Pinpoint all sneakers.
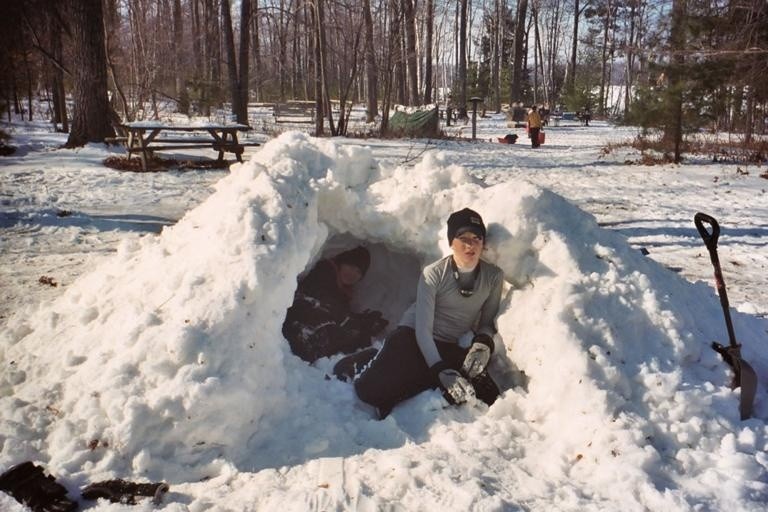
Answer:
[335,346,378,381]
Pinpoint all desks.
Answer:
[110,123,249,172]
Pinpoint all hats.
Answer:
[446,208,487,246]
[335,246,370,276]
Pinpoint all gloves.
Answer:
[353,311,388,341]
[461,335,494,379]
[430,361,477,405]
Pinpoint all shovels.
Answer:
[694,212,758,422]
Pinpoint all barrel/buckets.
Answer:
[538,127,545,143]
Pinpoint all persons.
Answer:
[339,204,522,417]
[585,106,592,126]
[445,96,455,127]
[527,105,543,149]
[281,242,389,359]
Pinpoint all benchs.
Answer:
[104,137,260,172]
